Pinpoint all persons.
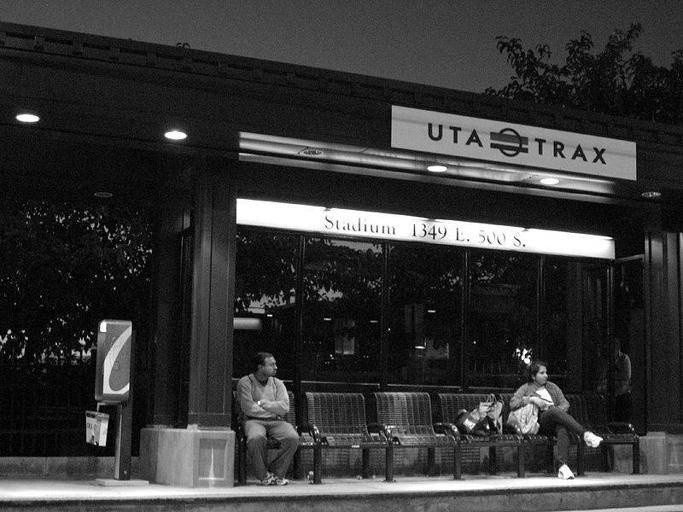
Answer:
[510,360,604,479]
[236,351,300,486]
[606,337,634,425]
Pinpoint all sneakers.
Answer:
[255,472,289,486]
[558,464,575,480]
[584,431,603,448]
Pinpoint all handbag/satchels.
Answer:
[456,393,504,436]
[507,403,540,435]
